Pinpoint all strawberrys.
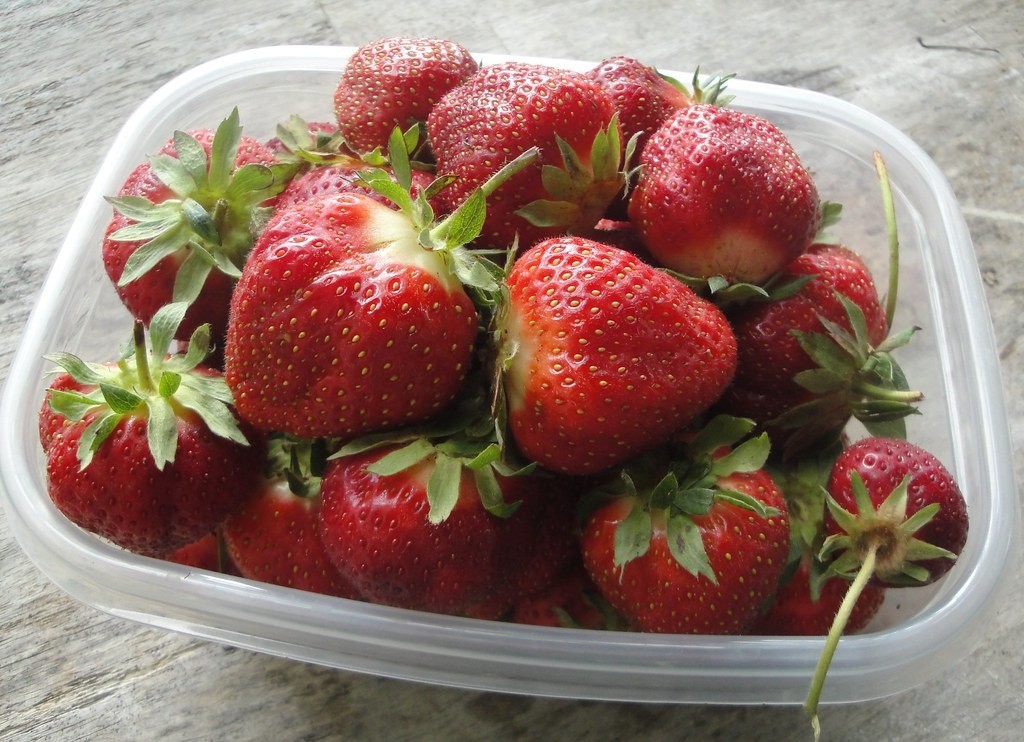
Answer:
[38,36,971,636]
